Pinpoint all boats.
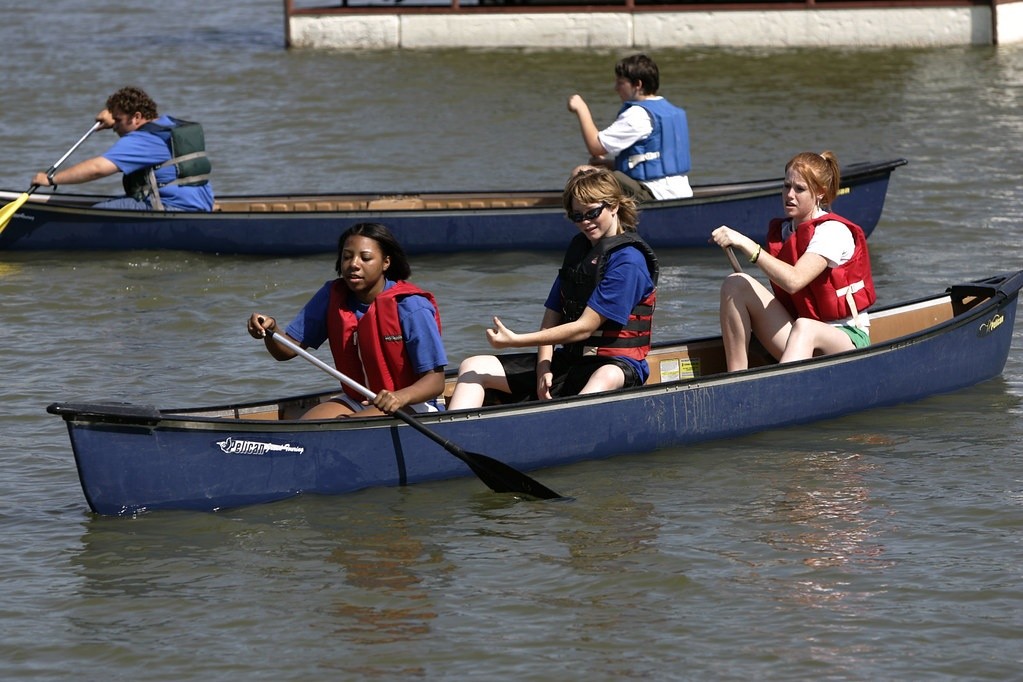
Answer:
[47,270,1023,512]
[1,159,908,254]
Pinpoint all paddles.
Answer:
[0,122,103,234]
[724,246,743,274]
[252,318,558,498]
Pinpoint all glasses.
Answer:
[568,202,607,223]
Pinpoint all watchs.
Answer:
[47,172,58,191]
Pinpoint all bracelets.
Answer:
[750,242,763,265]
[269,316,276,331]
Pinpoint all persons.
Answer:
[568,54,694,203]
[247,223,446,420]
[447,169,661,411]
[30,88,221,213]
[708,150,877,373]
